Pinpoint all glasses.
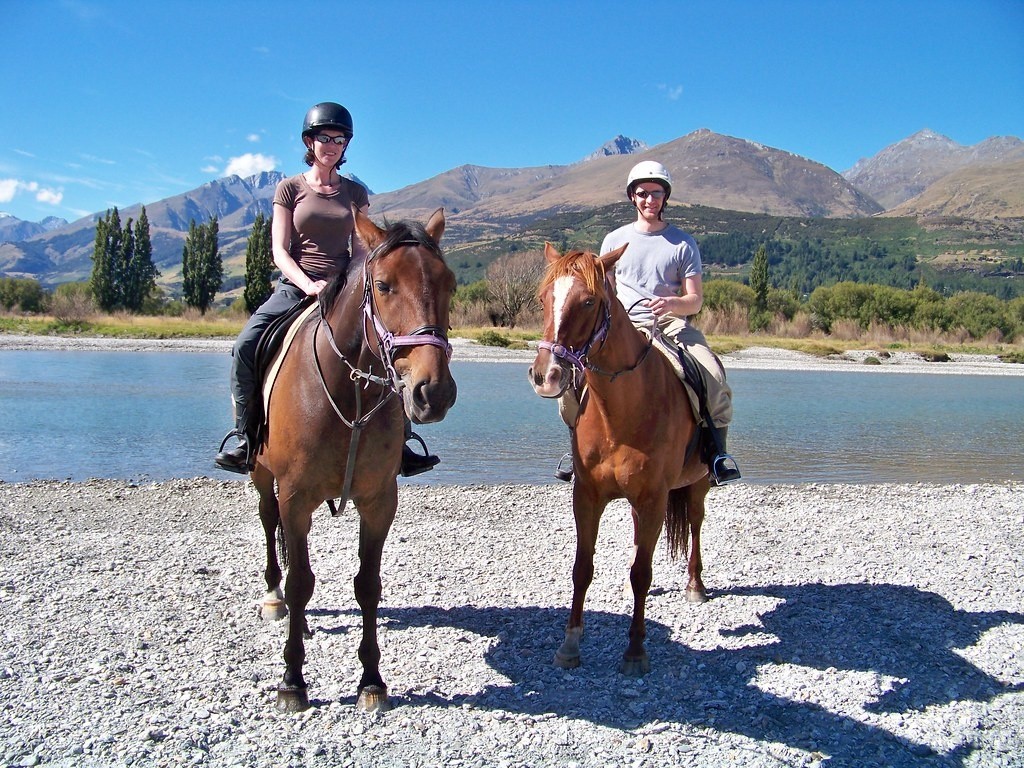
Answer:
[635,190,667,199]
[311,133,347,145]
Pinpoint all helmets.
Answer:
[628,161,672,199]
[301,102,354,139]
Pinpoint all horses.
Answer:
[527,240,728,674]
[232,201,458,716]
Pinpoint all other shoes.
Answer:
[708,461,735,479]
[216,440,254,466]
[402,449,440,474]
[555,460,574,480]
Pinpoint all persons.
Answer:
[216,101,440,477]
[554,161,740,483]
